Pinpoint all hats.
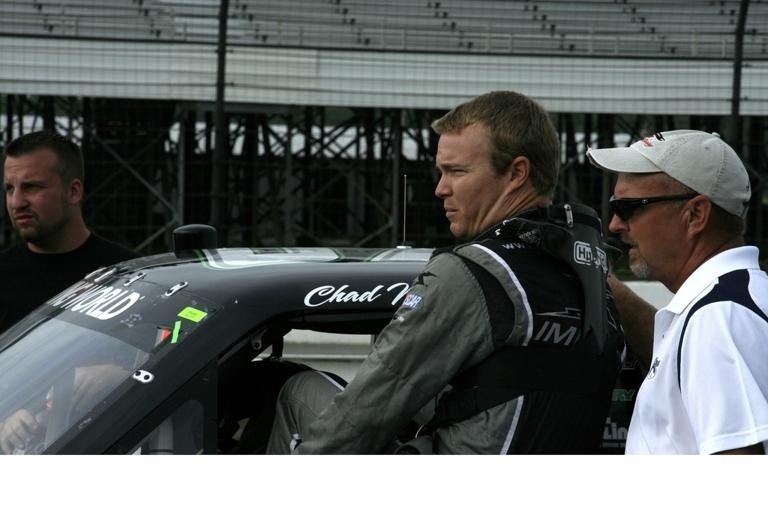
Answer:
[585,129,754,221]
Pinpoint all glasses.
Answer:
[606,192,700,223]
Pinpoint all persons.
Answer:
[0,131,142,455]
[584,125,767,455]
[266,89,624,454]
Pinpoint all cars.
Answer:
[0,225,646,454]
